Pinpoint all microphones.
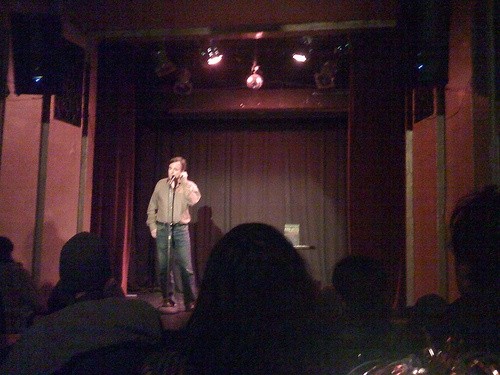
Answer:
[168,174,177,185]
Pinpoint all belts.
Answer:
[156,220,178,227]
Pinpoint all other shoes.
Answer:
[185,303,196,311]
[162,300,174,307]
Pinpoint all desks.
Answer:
[294,245,316,249]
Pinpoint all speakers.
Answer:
[397,0,450,85]
[12,12,66,95]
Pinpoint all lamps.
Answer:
[174,68,193,96]
[243,52,265,89]
[315,60,339,89]
[148,45,174,77]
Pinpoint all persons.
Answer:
[140,184,500,375]
[0,232,162,375]
[146,157,202,308]
[0,236,48,336]
